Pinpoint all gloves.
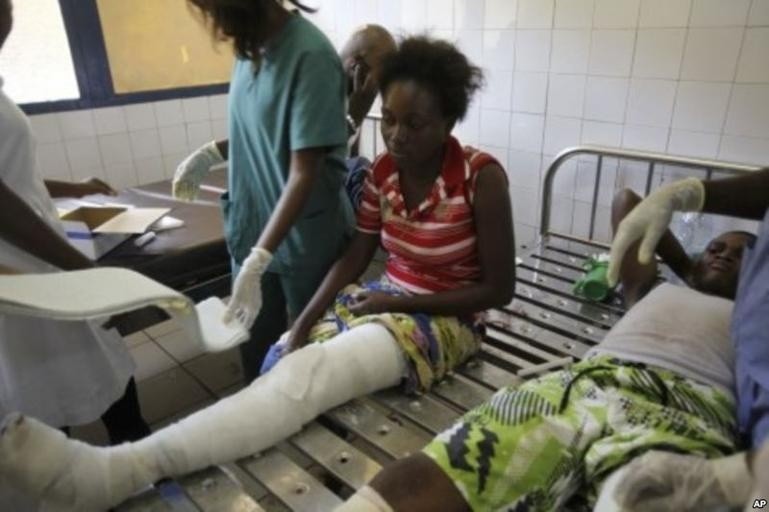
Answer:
[598,438,759,511]
[223,244,276,332]
[171,138,227,204]
[605,176,708,288]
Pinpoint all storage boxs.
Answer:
[54,200,172,263]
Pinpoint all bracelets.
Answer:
[343,112,359,134]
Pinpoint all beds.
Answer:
[108,145,768,512]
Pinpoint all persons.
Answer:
[335,24,399,212]
[588,165,769,510]
[181,0,360,385]
[0,0,155,443]
[1,33,518,512]
[330,187,759,511]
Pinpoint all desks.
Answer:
[55,166,234,338]
